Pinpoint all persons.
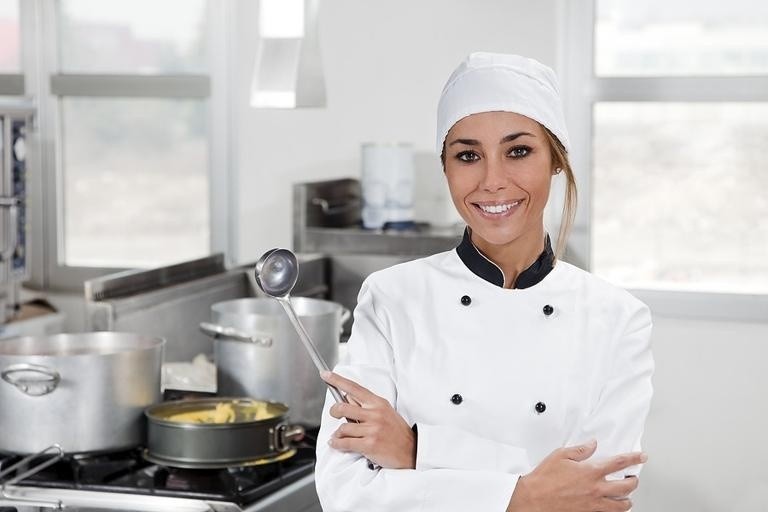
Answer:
[313,53,654,512]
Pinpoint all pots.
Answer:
[200,296,349,429]
[143,395,303,470]
[1,330,167,455]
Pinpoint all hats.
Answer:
[436,51,570,158]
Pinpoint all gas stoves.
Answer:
[7,449,318,512]
